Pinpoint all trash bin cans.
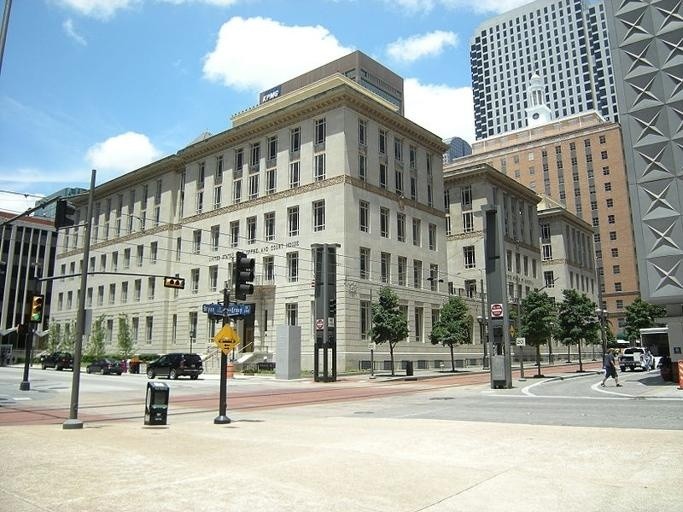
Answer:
[407,361,413,375]
[144,381,169,425]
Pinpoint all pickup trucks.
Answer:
[618,346,655,371]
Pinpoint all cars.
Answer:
[41,352,204,380]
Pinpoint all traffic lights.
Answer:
[235,251,255,301]
[29,294,44,323]
[328,297,336,317]
[164,276,184,289]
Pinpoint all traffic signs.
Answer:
[202,304,251,320]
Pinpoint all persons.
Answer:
[601,348,623,387]
[640,347,652,372]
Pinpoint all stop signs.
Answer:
[491,303,504,317]
[315,319,324,330]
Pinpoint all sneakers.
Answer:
[616,384,621,386]
[601,383,606,387]
